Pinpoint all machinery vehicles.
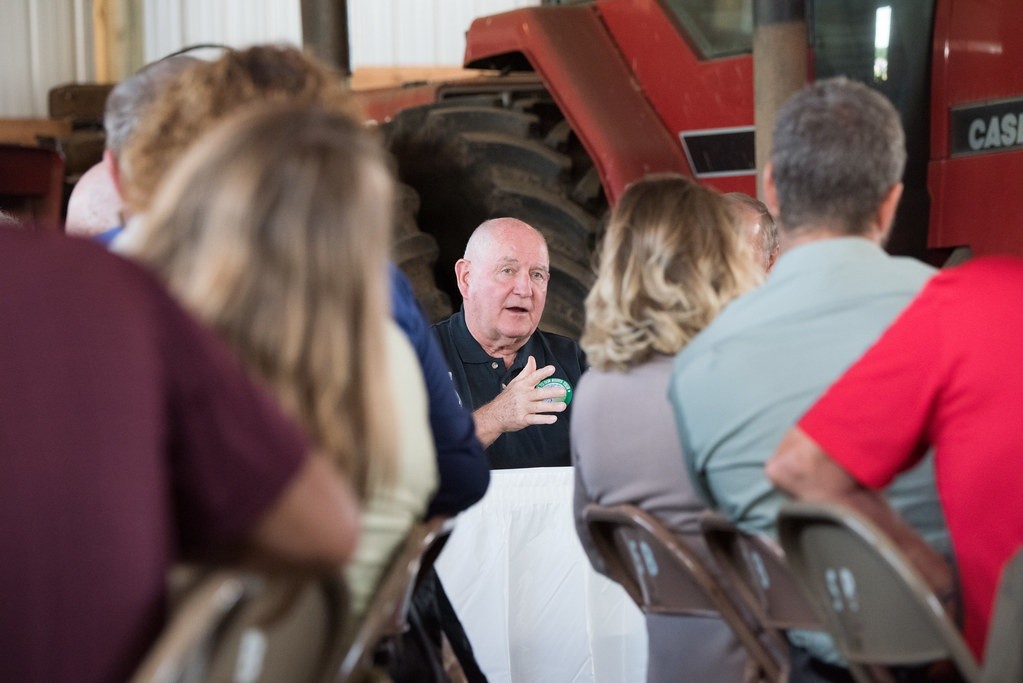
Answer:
[380,0,1023,333]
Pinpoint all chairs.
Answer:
[127,513,489,683]
[581,496,988,683]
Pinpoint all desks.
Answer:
[432,466,650,683]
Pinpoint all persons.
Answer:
[0,42,1023,683]
